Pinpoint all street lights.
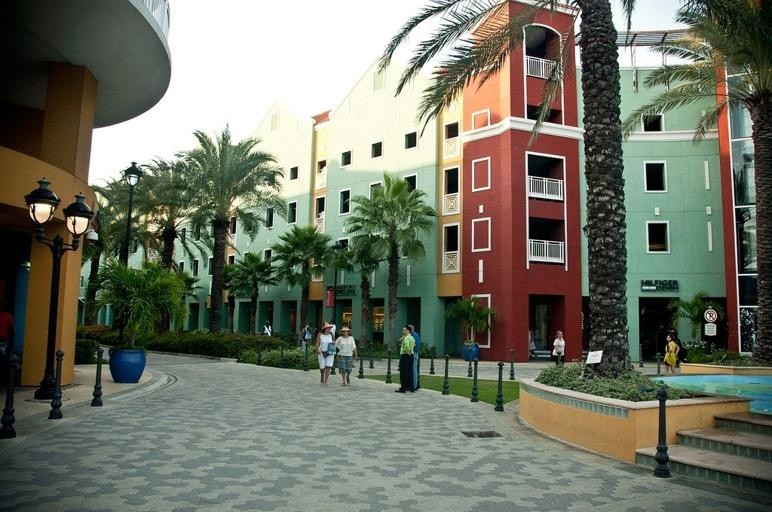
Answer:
[24,175,96,399]
[117,160,144,345]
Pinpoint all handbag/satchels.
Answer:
[328,343,336,355]
[322,351,330,358]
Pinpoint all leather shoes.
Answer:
[395,386,421,393]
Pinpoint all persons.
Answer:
[330,319,336,337]
[301,321,313,346]
[263,320,271,335]
[528,328,537,360]
[663,333,680,376]
[336,326,359,386]
[394,325,416,393]
[408,325,420,391]
[316,321,336,385]
[552,330,565,365]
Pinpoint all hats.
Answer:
[340,326,352,335]
[321,323,334,331]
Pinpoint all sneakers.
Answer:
[320,376,350,386]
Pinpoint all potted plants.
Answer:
[82,253,185,383]
